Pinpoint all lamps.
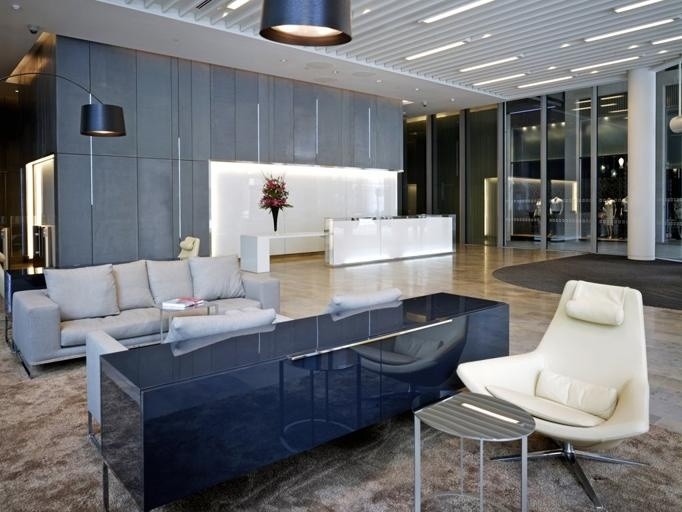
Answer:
[0,72,126,138]
[669,61,682,134]
[258,0,352,48]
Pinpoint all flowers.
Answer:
[258,172,294,214]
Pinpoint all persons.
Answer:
[534,202,544,234]
[603,198,617,238]
[620,197,628,240]
[547,196,563,239]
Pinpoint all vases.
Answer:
[270,206,279,232]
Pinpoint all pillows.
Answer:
[111,259,156,311]
[324,288,402,315]
[188,255,247,301]
[145,259,194,305]
[43,263,120,322]
[162,308,277,345]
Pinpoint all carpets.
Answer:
[1,313,681,512]
[491,252,682,313]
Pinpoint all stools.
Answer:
[412,392,537,512]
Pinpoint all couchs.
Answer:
[4,255,281,379]
[84,291,510,512]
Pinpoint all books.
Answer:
[161,298,204,311]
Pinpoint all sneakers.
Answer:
[608,236,612,239]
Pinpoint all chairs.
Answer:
[455,279,650,510]
